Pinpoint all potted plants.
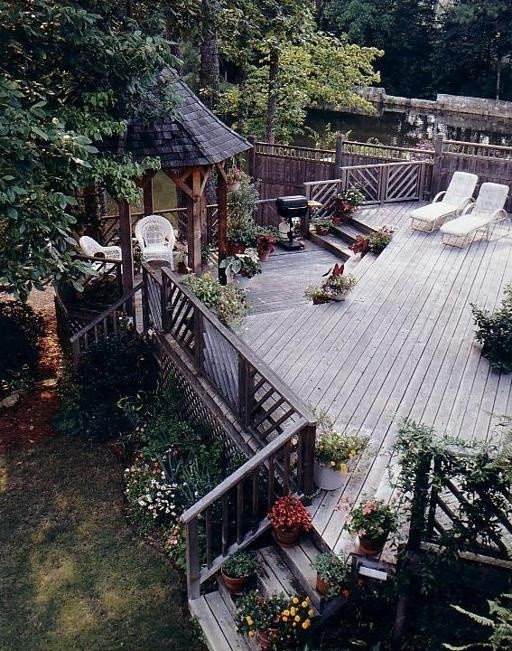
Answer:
[177,186,392,307]
[313,551,350,599]
[268,495,312,548]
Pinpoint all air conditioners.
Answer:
[221,550,263,592]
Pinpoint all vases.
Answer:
[314,462,347,490]
[358,531,389,555]
[256,629,276,647]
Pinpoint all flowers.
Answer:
[233,588,317,639]
[346,498,400,538]
[315,431,362,474]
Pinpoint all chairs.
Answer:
[79,235,123,276]
[409,171,478,234]
[135,214,176,274]
[440,182,511,249]
